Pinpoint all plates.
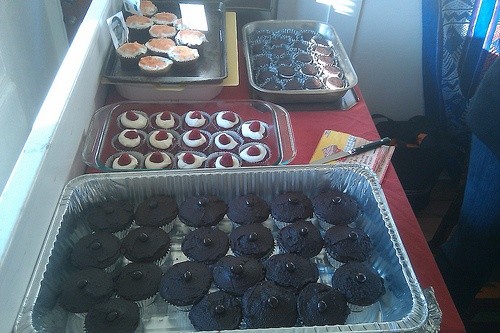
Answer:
[98,1,230,83]
[13,163,430,331]
[79,98,299,168]
[241,19,359,105]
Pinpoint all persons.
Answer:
[111,16,127,49]
[428,56,500,316]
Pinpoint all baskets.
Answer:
[372,114,444,211]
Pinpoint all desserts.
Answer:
[62,191,384,333]
[112,109,268,169]
[116,0,209,77]
[251,29,349,91]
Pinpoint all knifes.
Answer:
[308,137,395,163]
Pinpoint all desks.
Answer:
[84,39,466,333]
